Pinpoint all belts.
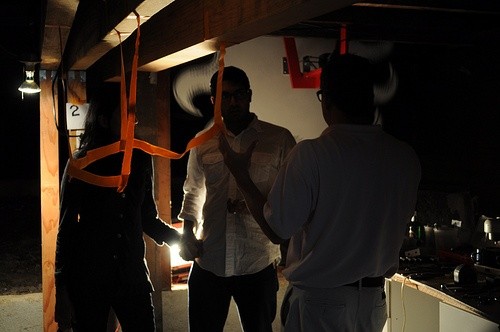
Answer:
[344,276,384,288]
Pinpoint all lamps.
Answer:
[18,61,42,94]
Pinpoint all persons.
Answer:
[218,53,418,332]
[55,82,202,332]
[177,66,296,332]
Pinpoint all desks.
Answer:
[384,247,500,332]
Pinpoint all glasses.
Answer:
[216,88,250,100]
[316,85,334,102]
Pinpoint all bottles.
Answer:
[399,209,426,280]
